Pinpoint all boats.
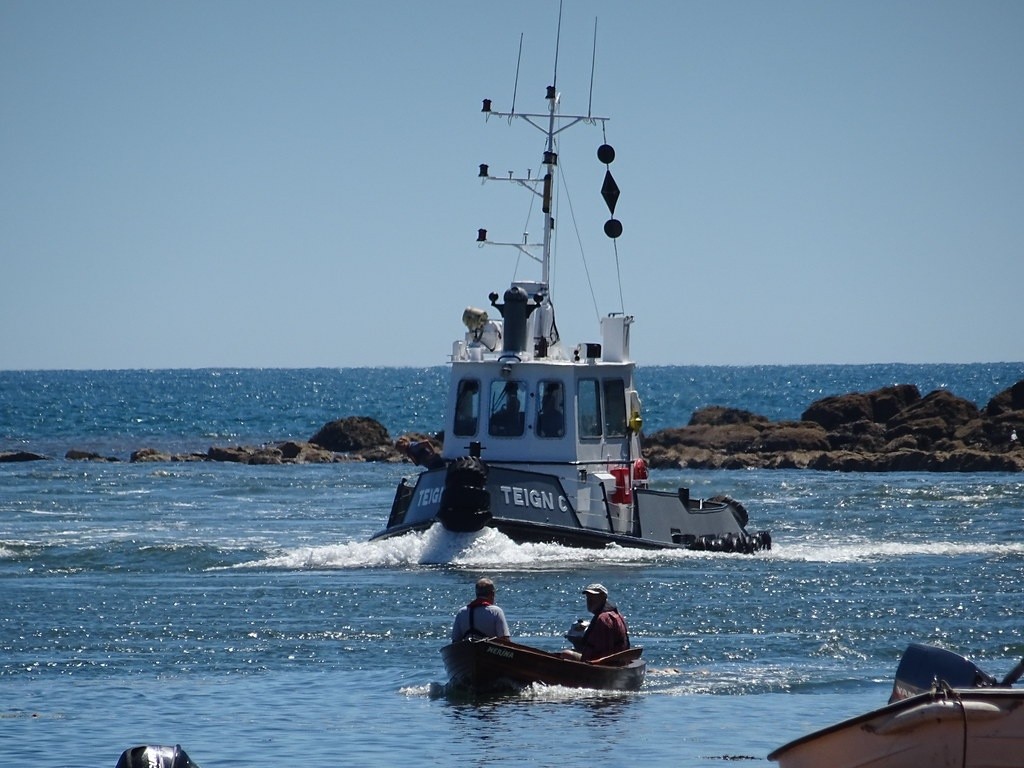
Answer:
[766,685,1023,768]
[367,2,773,554]
[440,634,647,695]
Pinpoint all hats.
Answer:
[583,584,609,596]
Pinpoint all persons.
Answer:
[537,394,563,438]
[449,578,514,650]
[490,397,525,438]
[548,583,631,667]
[394,437,447,471]
